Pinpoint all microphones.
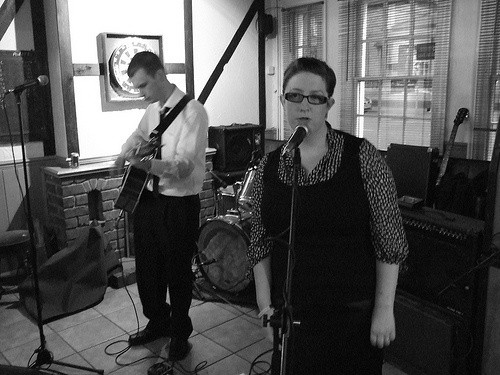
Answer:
[200,257,217,265]
[283,125,308,157]
[5,74,49,94]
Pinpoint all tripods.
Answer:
[13,90,105,375]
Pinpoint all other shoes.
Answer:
[129,327,168,346]
[169,336,189,360]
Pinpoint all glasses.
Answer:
[285,93,326,105]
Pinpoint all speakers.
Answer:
[395,217,483,311]
[207,124,264,173]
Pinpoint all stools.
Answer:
[0,230,30,302]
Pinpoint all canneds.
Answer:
[71,152,79,168]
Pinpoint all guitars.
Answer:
[435,107,469,185]
[114,131,161,214]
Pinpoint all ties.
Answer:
[152,107,170,198]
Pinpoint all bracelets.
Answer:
[258,304,274,318]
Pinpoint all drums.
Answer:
[196,208,253,293]
[217,184,235,218]
[239,167,258,213]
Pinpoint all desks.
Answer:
[213,171,246,216]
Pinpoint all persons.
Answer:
[247,57,409,375]
[115,51,208,361]
